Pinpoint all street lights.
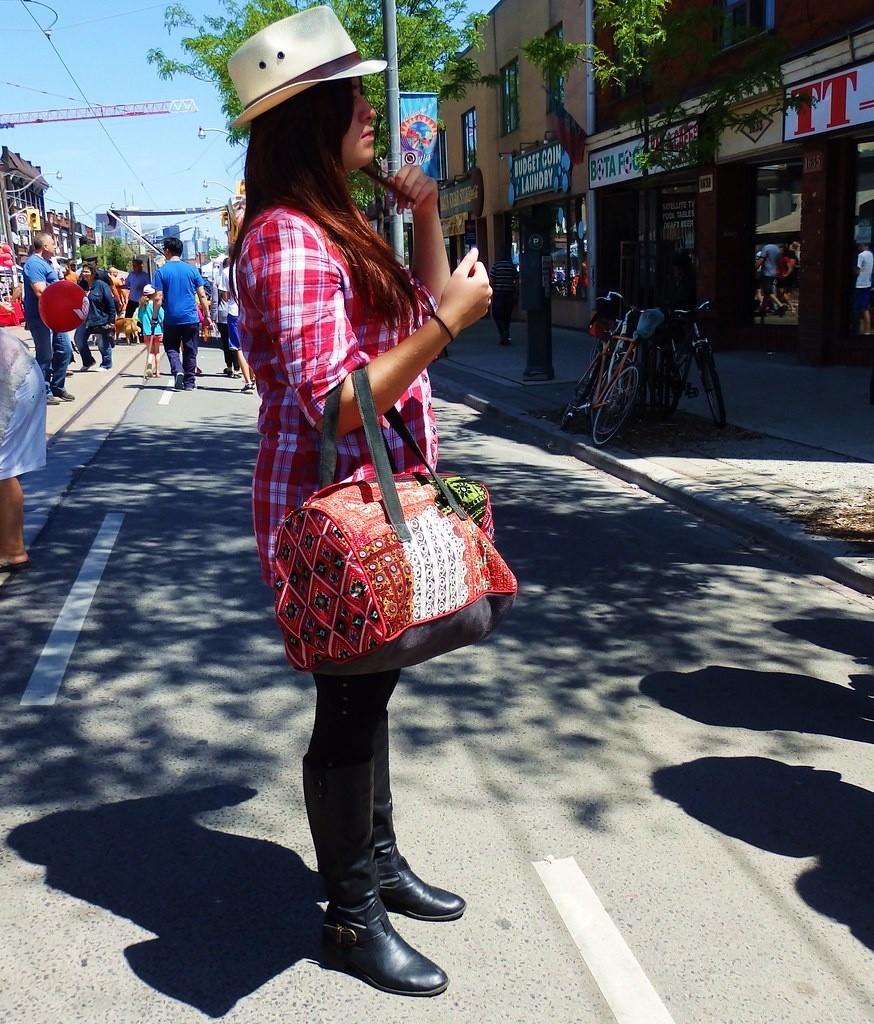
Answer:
[0,169,63,294]
[69,200,116,272]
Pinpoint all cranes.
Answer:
[0,80,201,128]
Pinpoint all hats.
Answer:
[143,284,156,295]
[227,5,388,127]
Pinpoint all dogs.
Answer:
[114,317,141,345]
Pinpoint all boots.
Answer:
[302,749,450,997]
[374,708,467,921]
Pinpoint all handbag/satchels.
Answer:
[274,367,518,676]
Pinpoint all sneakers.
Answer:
[53,391,75,400]
[47,394,60,405]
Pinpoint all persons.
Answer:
[224,6,494,997]
[754,238,799,317]
[489,245,519,345]
[854,234,874,335]
[0,327,46,574]
[552,259,588,296]
[11,231,255,405]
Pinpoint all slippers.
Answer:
[0,561,30,573]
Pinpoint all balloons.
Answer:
[38,281,90,333]
[0,245,13,268]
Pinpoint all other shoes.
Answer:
[81,360,96,371]
[175,372,184,389]
[186,387,196,391]
[500,336,512,345]
[251,380,255,385]
[234,374,241,378]
[224,368,233,376]
[96,367,112,371]
[241,384,255,394]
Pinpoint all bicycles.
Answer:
[560,290,727,449]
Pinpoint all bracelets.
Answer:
[152,316,158,321]
[432,315,454,341]
